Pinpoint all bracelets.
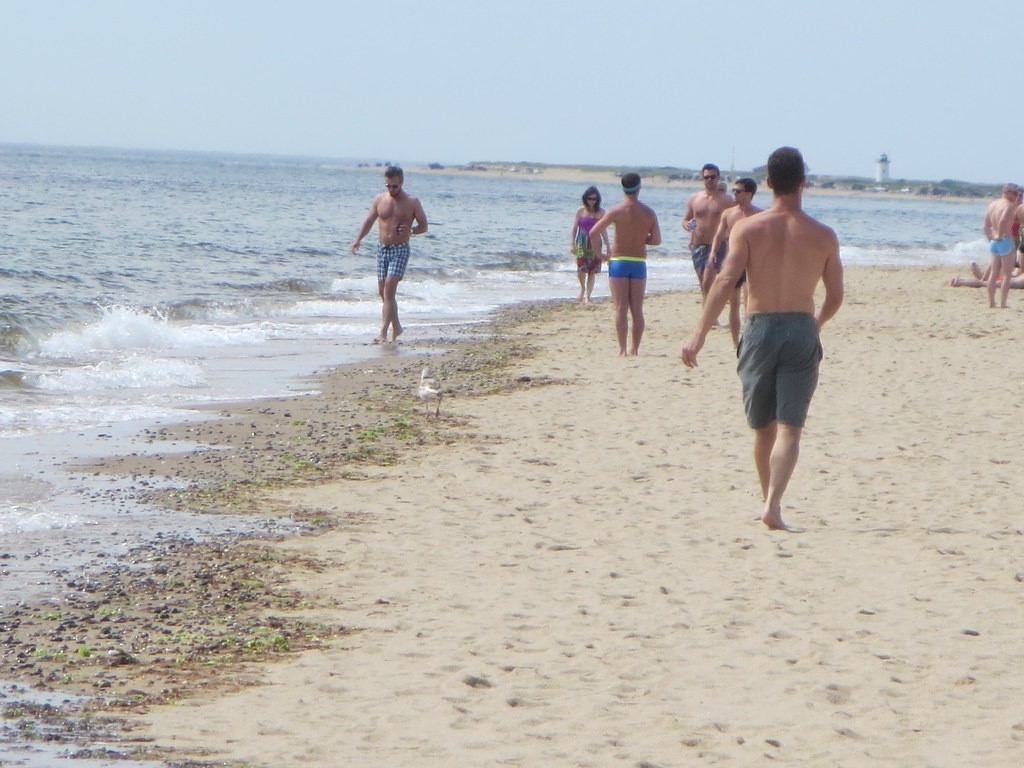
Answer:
[408,227,414,237]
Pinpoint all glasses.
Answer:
[732,189,745,193]
[386,181,401,188]
[588,198,598,201]
[1009,190,1018,195]
[704,175,717,179]
[1019,194,1023,197]
[719,189,726,192]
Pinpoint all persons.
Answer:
[570,186,611,303]
[589,172,662,356]
[352,165,429,342]
[679,146,845,530]
[949,182,1024,309]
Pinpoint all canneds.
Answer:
[689,219,696,231]
